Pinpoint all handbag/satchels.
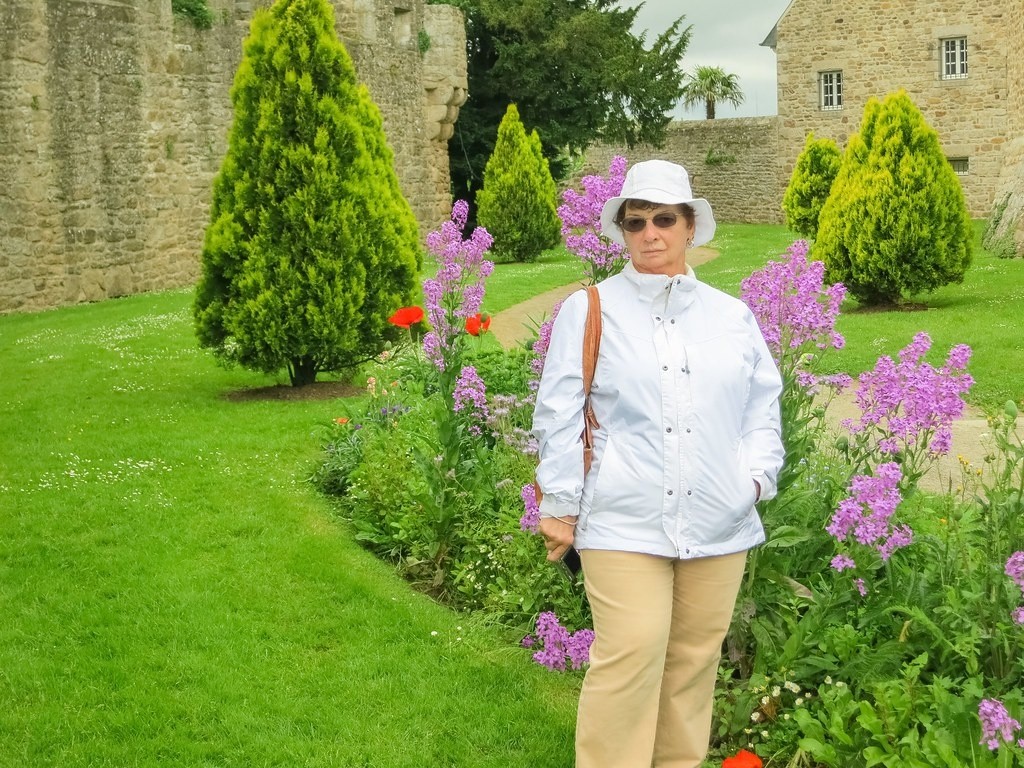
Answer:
[534,285,599,509]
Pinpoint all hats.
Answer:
[599,159,716,247]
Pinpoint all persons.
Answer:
[532,159,786,768]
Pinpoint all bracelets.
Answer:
[540,511,579,526]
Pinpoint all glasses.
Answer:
[620,213,688,232]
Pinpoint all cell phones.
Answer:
[561,546,582,578]
